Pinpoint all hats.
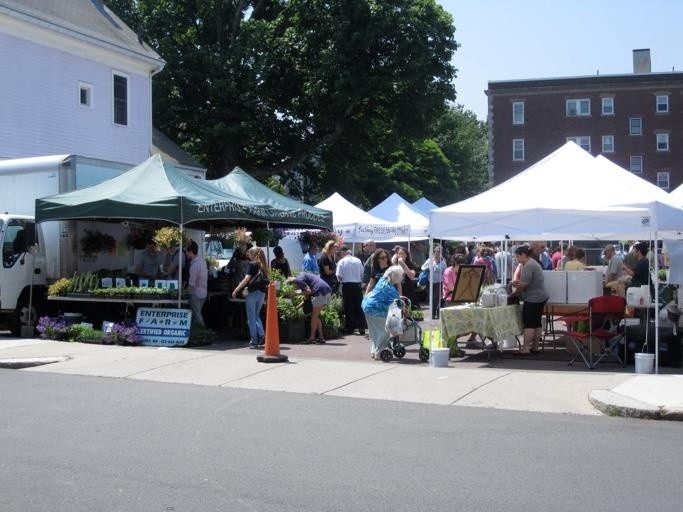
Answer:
[391,245,400,251]
[601,245,614,256]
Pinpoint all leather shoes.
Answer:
[342,327,365,335]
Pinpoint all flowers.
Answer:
[88,287,168,295]
[112,322,142,344]
[47,278,71,296]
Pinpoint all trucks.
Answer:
[202,231,305,280]
[0,150,210,336]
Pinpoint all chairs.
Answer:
[558,295,627,369]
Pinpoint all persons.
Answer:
[135,229,655,361]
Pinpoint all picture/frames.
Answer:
[450,264,486,303]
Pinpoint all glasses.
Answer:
[538,245,544,249]
[377,255,387,260]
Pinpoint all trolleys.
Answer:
[378,295,431,364]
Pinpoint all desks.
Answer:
[541,303,590,354]
[438,301,525,368]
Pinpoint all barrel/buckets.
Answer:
[430,348,450,367]
[634,352,655,374]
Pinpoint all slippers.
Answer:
[512,348,540,356]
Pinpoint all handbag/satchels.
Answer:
[246,267,269,292]
[417,270,428,285]
[302,297,312,314]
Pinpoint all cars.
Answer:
[597,244,622,266]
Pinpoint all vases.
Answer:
[62,287,67,297]
[89,294,171,300]
[58,290,61,296]
[118,337,129,346]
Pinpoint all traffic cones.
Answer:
[251,281,293,365]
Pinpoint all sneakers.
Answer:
[371,353,381,361]
[431,314,439,320]
[248,335,265,349]
[449,339,497,357]
